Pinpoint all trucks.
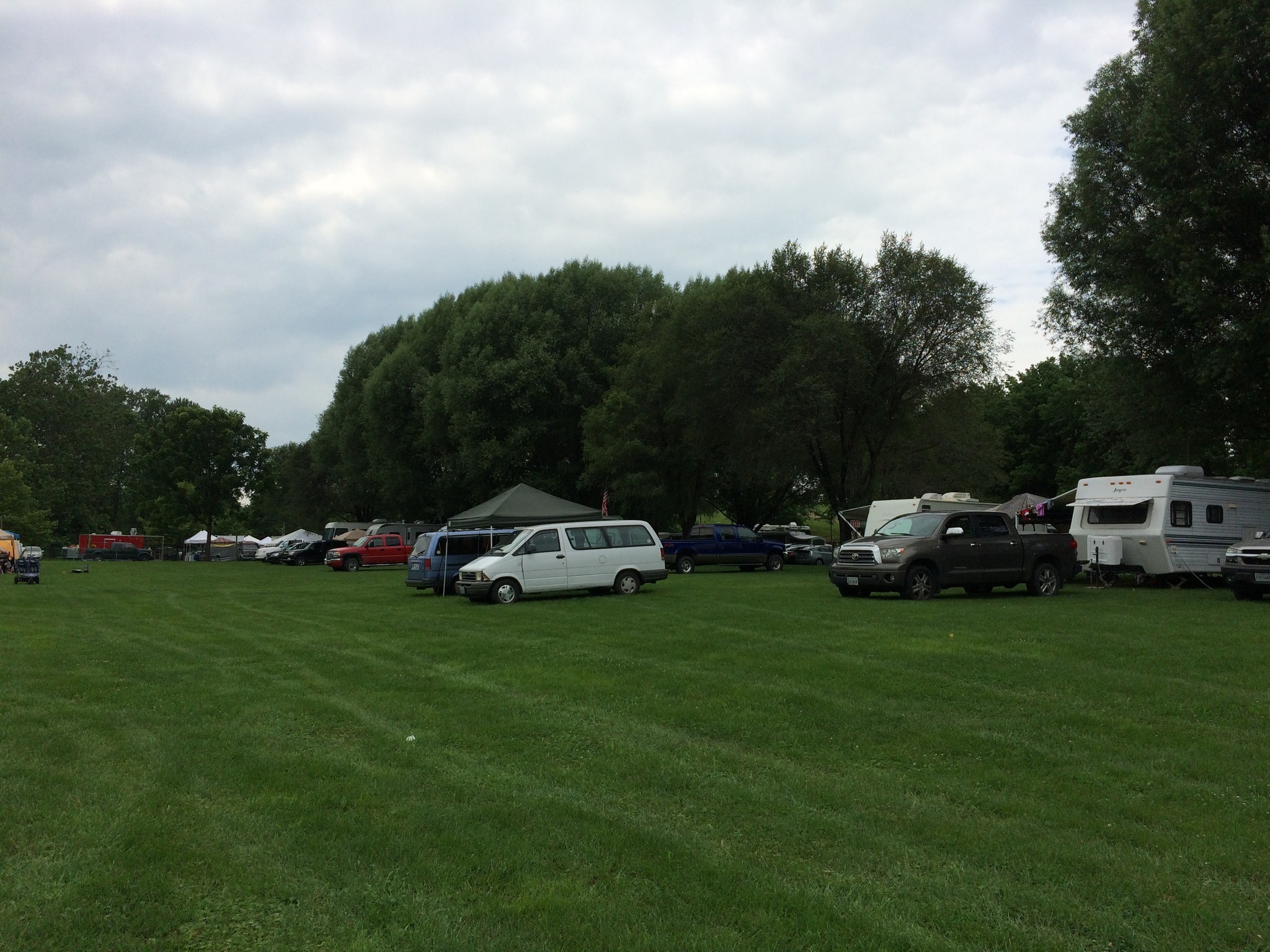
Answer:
[838,491,1048,538]
[321,518,407,546]
[1015,465,1270,588]
[514,515,633,549]
[365,519,496,547]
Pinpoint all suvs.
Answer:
[454,519,669,604]
[234,538,350,566]
[405,527,519,596]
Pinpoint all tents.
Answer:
[442,483,602,597]
[0,528,20,572]
[184,529,323,556]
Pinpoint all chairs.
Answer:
[575,534,585,548]
[0,560,3,574]
[538,537,553,549]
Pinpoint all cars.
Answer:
[752,521,837,566]
[23,546,44,561]
[1220,528,1270,600]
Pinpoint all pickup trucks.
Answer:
[828,508,1084,601]
[660,524,788,574]
[324,534,414,572]
[83,542,152,562]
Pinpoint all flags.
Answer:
[602,480,609,515]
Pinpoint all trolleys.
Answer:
[14,557,41,585]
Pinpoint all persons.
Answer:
[332,527,366,541]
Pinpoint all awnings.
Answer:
[1067,497,1153,506]
[839,504,871,537]
